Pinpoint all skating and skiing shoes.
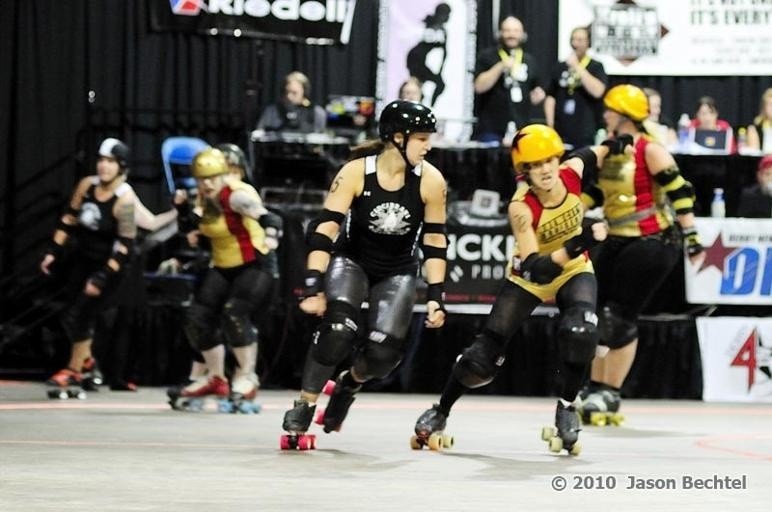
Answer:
[110,376,137,393]
[232,371,262,415]
[542,401,584,458]
[280,397,316,451]
[579,386,626,427]
[573,378,593,405]
[315,370,364,434]
[175,376,193,389]
[411,404,456,451]
[46,367,88,401]
[82,355,103,394]
[167,373,235,414]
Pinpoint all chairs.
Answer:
[161,135,211,196]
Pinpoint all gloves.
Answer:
[175,211,203,235]
[36,236,66,268]
[263,229,281,252]
[188,226,211,253]
[425,281,449,317]
[601,131,636,155]
[680,227,705,259]
[168,193,194,216]
[564,221,600,260]
[90,264,118,294]
[294,266,326,306]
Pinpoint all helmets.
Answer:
[510,122,566,175]
[96,135,130,161]
[377,100,437,142]
[214,142,247,167]
[190,147,229,177]
[603,83,652,124]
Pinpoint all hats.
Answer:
[755,156,772,171]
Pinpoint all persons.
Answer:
[282,101,448,453]
[397,78,424,100]
[412,123,637,456]
[111,185,177,391]
[408,3,450,107]
[642,87,677,143]
[736,157,772,218]
[473,16,546,144]
[688,95,736,154]
[256,71,327,133]
[744,89,772,155]
[40,136,135,399]
[576,84,708,426]
[543,26,607,150]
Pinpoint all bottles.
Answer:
[711,188,727,219]
[679,113,690,143]
